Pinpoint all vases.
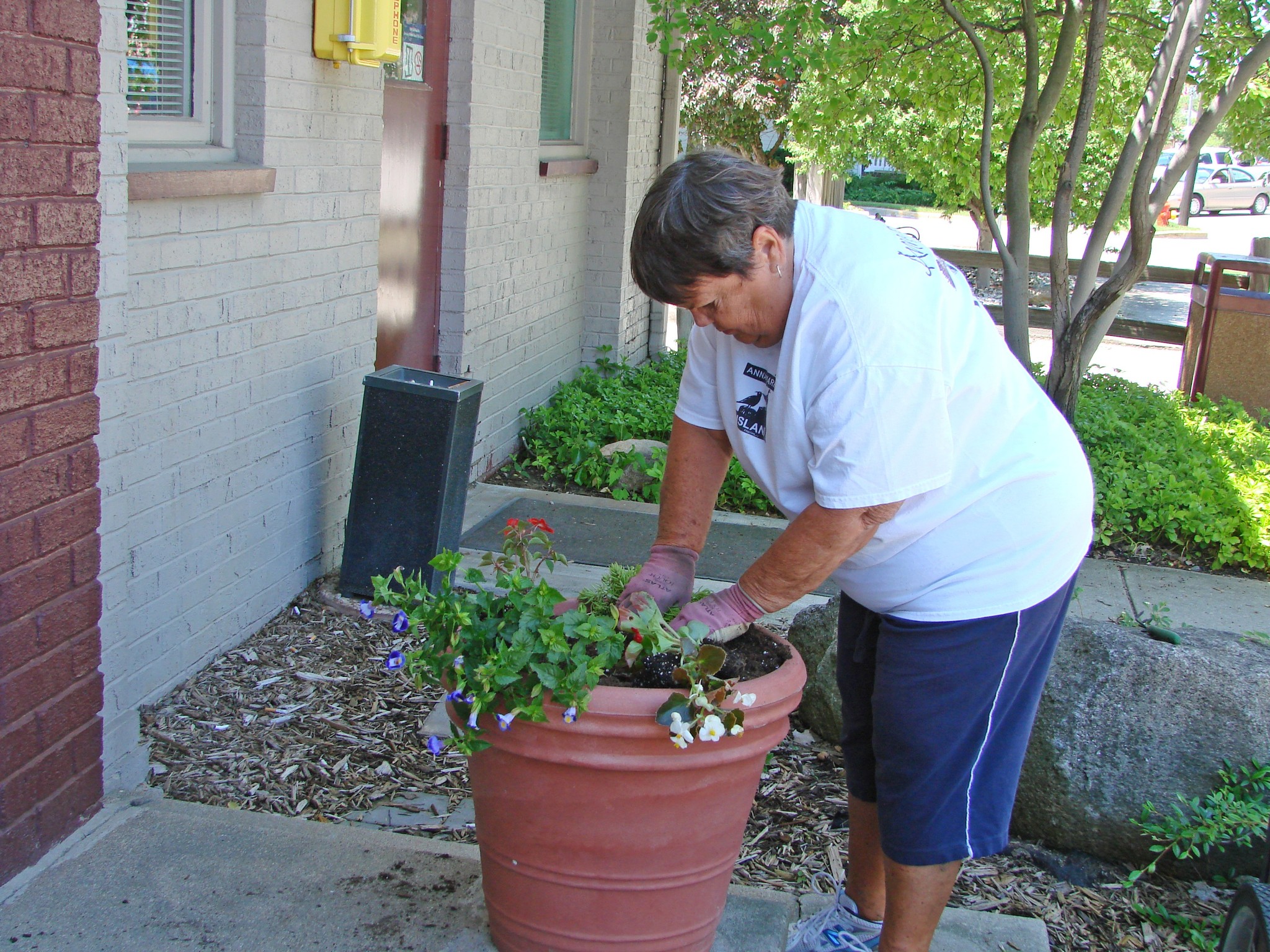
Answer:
[439,595,808,952]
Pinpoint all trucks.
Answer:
[1156,143,1248,164]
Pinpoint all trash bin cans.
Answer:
[337,363,485,612]
[1175,251,1270,430]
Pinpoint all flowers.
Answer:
[356,516,758,755]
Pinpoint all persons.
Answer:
[609,151,1099,952]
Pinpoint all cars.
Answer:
[1146,163,1270,218]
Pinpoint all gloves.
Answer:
[664,579,770,643]
[614,544,699,628]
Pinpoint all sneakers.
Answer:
[784,869,884,952]
